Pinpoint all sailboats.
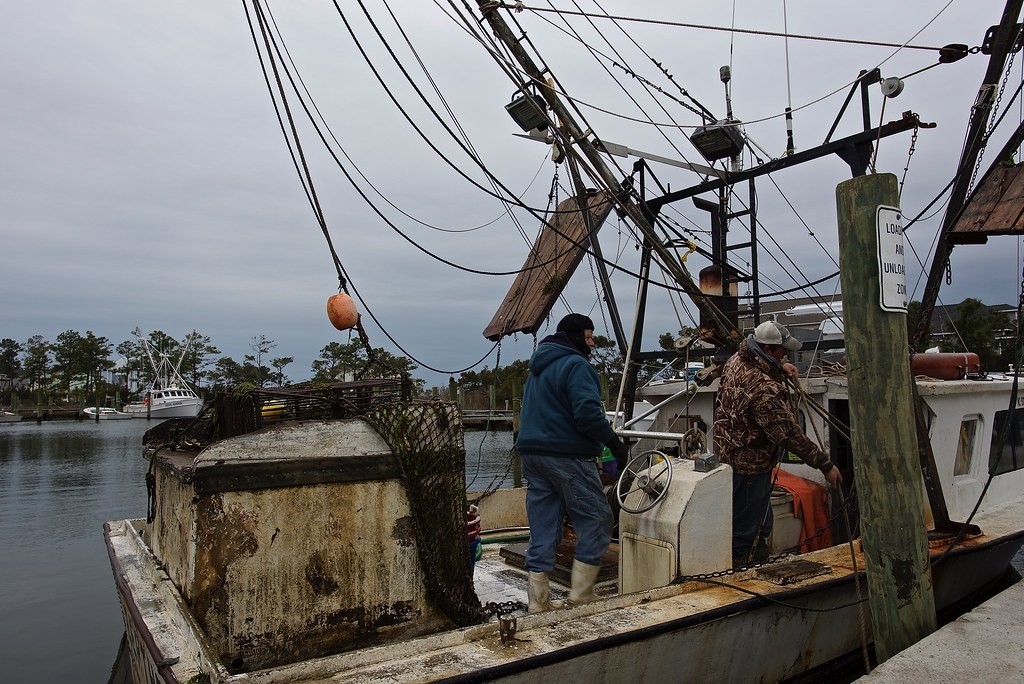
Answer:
[102,0,1024,684]
[123,325,205,419]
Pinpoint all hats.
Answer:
[556,314,595,355]
[753,321,803,351]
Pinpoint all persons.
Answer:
[711,318,843,566]
[516,311,633,614]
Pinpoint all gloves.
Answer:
[604,434,629,471]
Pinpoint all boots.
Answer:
[566,558,602,604]
[527,570,564,615]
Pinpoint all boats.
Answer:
[83,407,133,420]
[0,411,23,422]
